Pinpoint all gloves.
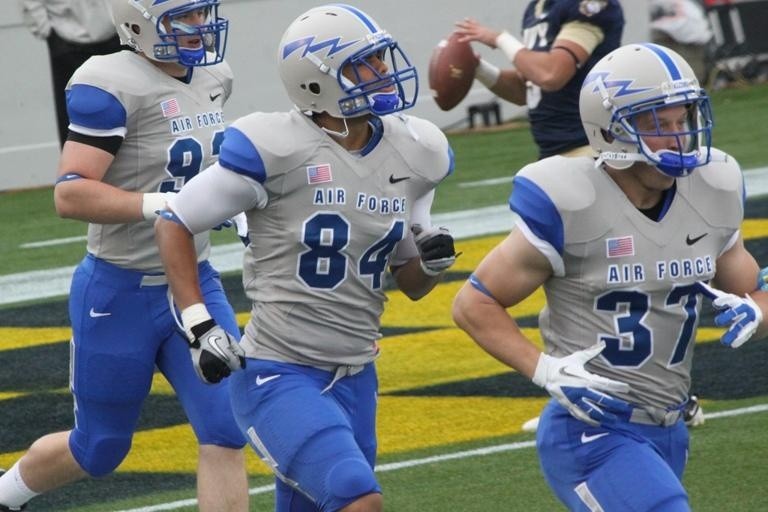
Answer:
[696,280,764,349]
[185,317,246,384]
[410,223,456,277]
[532,340,630,428]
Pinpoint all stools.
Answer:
[469,103,502,128]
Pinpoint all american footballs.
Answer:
[426,32,476,113]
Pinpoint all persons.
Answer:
[453,42,767,512]
[21,0,121,155]
[0,0,248,512]
[153,2,458,511]
[452,0,625,437]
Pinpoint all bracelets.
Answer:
[495,31,526,64]
[472,59,502,90]
[141,192,166,221]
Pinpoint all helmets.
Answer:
[277,4,418,119]
[114,0,229,67]
[578,43,714,178]
